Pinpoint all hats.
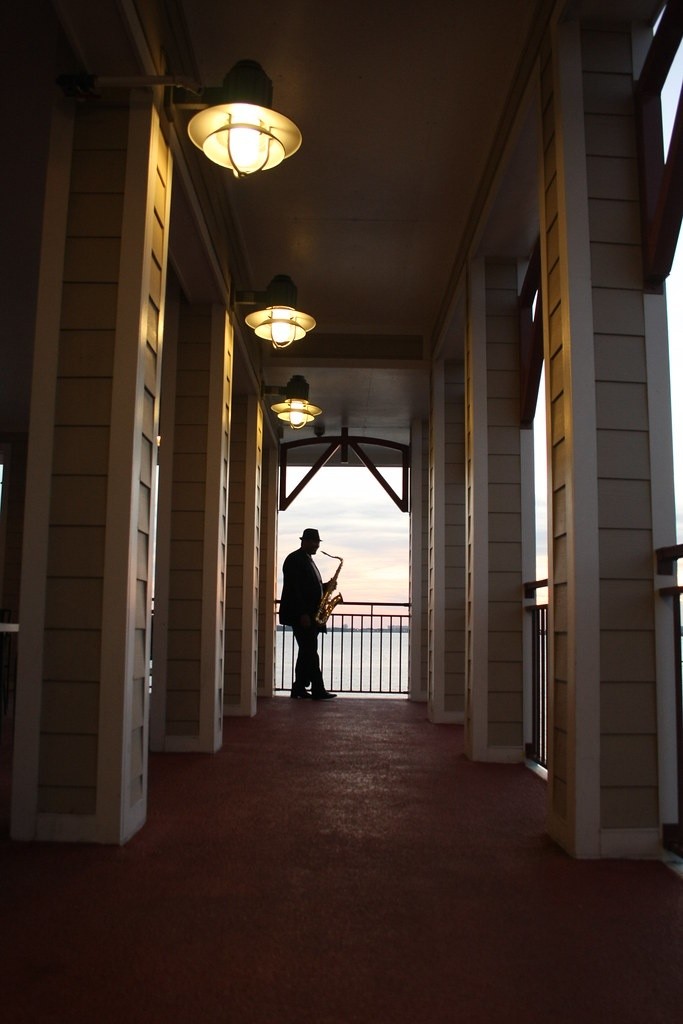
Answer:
[299,529,321,541]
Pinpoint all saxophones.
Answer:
[314,550,346,626]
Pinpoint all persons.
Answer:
[279,528,338,700]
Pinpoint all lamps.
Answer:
[259,374,323,429]
[163,59,303,180]
[229,273,318,351]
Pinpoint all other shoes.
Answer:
[291,682,312,699]
[312,687,337,700]
